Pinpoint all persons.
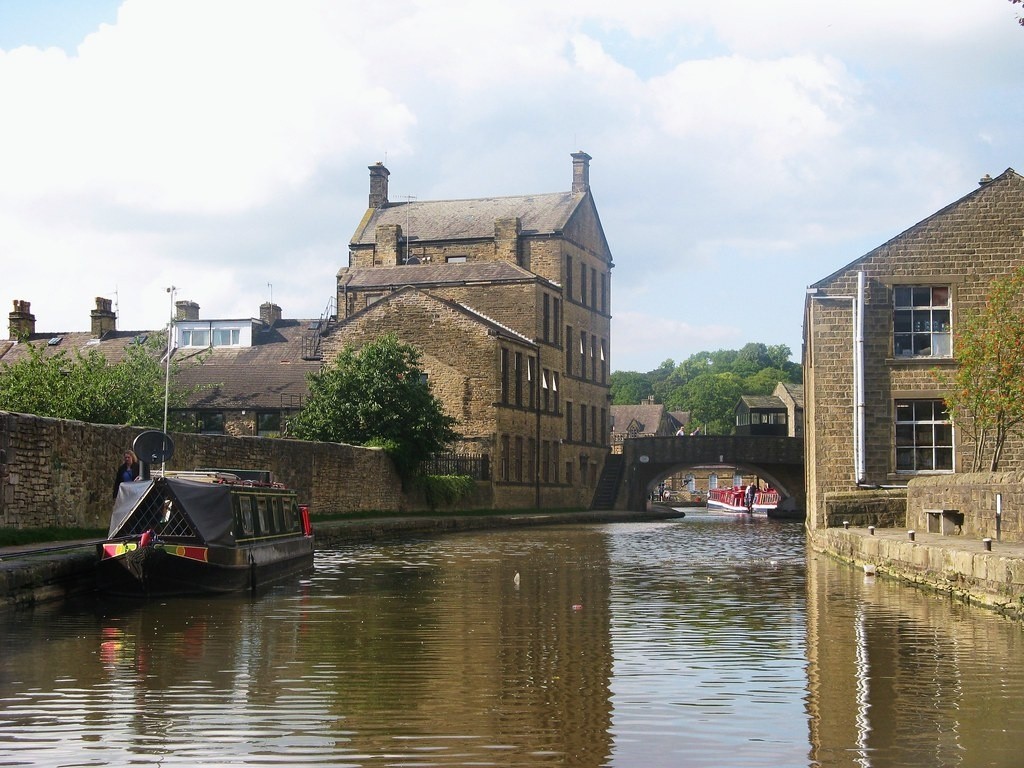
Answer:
[659,487,664,500]
[690,430,696,435]
[112,450,140,501]
[745,481,756,506]
[676,426,685,436]
[694,427,700,435]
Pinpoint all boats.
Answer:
[652,490,711,507]
[95,467,316,594]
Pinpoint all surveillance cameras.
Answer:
[806,288,818,294]
